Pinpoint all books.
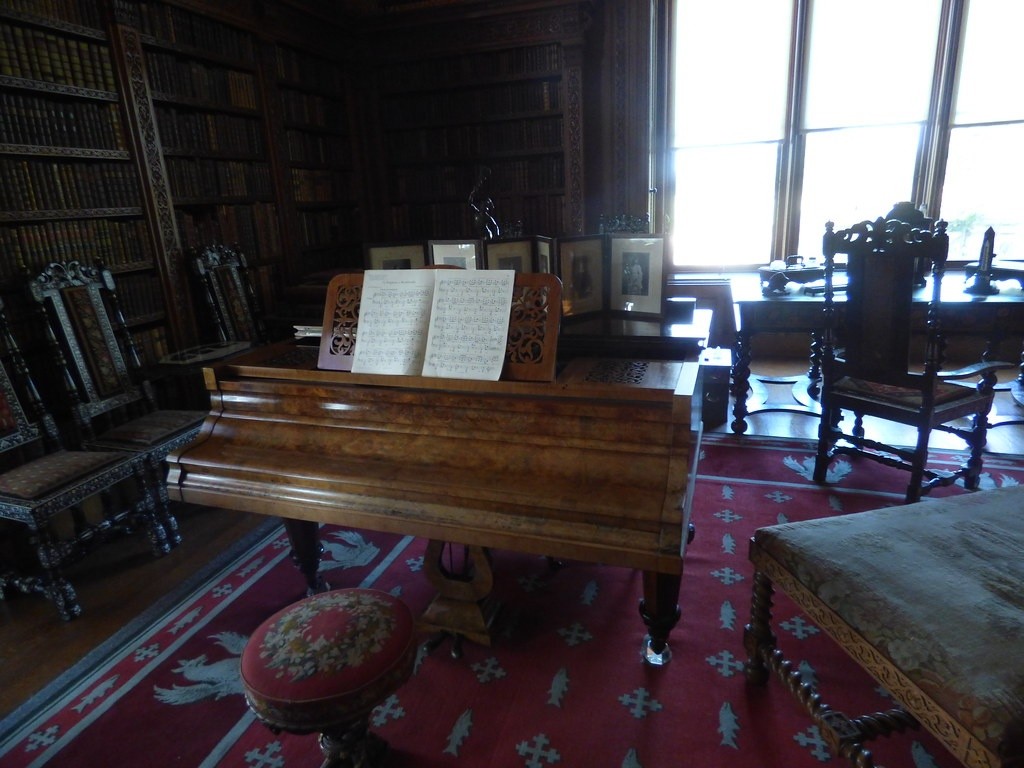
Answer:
[376,42,571,242]
[351,269,516,381]
[0,3,359,405]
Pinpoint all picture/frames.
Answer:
[553,235,607,320]
[533,234,553,274]
[427,239,482,270]
[483,236,533,274]
[606,233,666,318]
[363,239,427,269]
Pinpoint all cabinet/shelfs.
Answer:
[0,1,661,392]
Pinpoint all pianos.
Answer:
[164,262,708,673]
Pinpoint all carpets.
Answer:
[0,433,1020,768]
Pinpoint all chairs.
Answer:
[742,483,1024,768]
[8,259,211,549]
[188,240,275,355]
[0,271,172,624]
[812,215,1015,505]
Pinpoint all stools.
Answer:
[239,587,416,767]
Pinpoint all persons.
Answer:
[623,252,647,295]
[571,256,592,300]
